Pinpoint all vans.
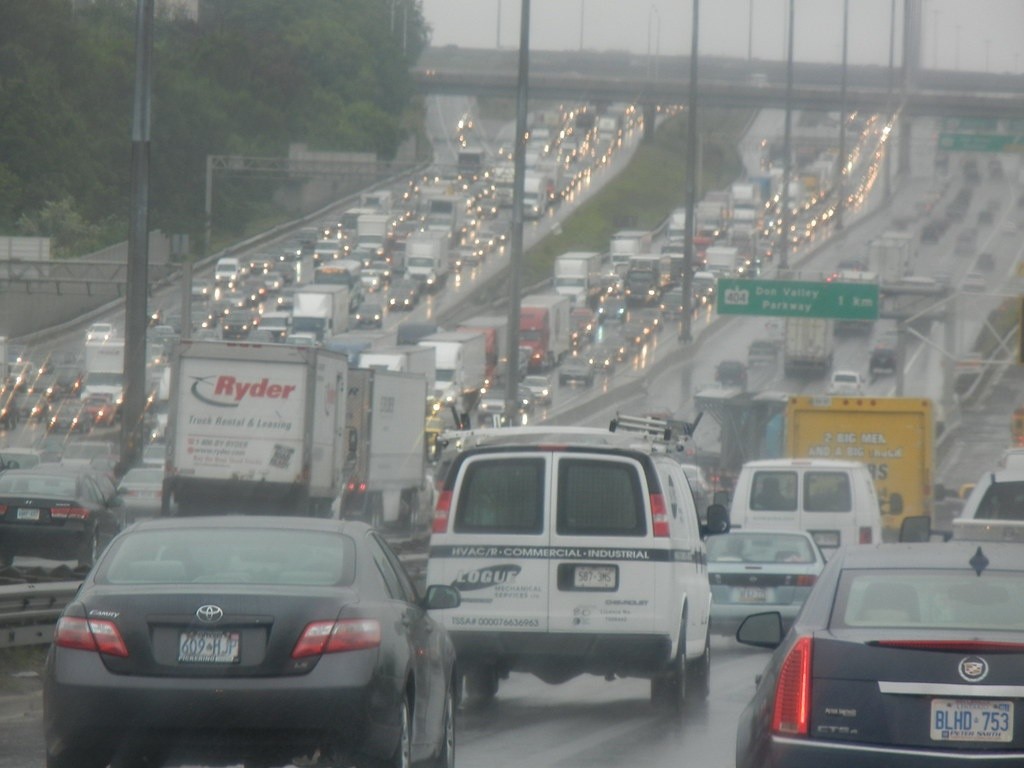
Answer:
[424,428,713,712]
[728,457,883,564]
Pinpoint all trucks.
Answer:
[518,295,570,371]
[356,214,393,259]
[457,317,511,390]
[290,283,348,342]
[609,230,652,277]
[81,339,125,407]
[403,231,449,293]
[342,367,440,532]
[418,331,485,416]
[156,337,357,523]
[426,194,467,246]
[553,252,601,311]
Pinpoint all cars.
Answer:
[957,468,1024,520]
[735,541,1024,768]
[700,528,828,638]
[0,465,125,567]
[0,79,1024,519]
[43,515,461,768]
